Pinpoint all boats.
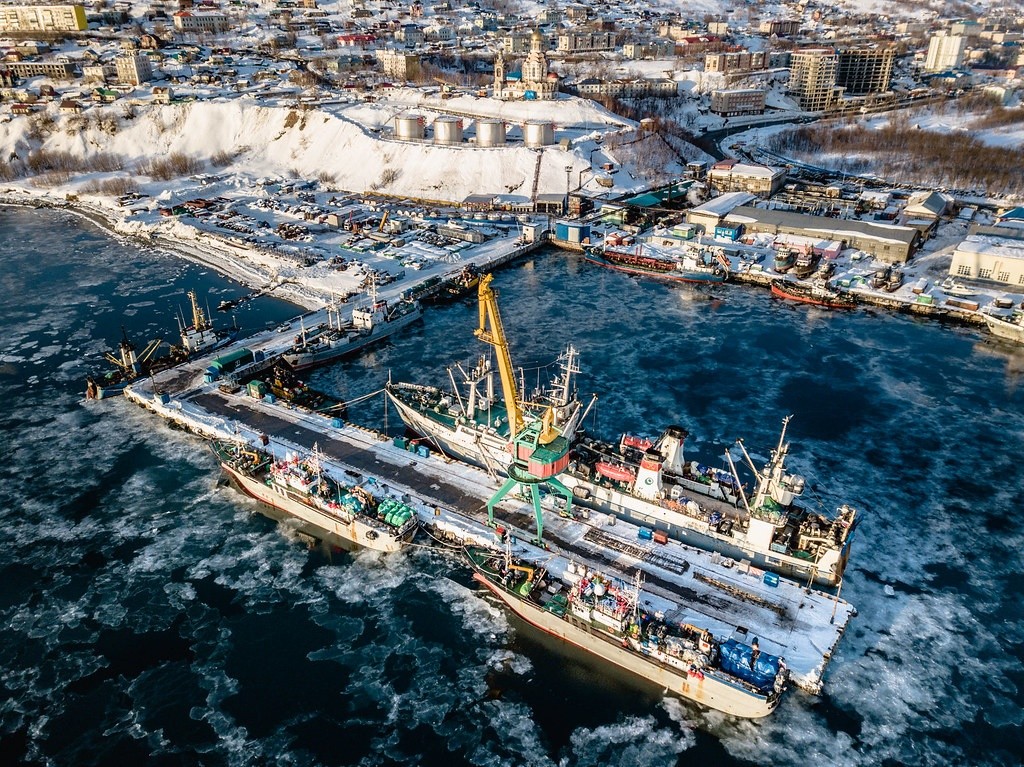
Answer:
[85,290,243,402]
[770,273,857,310]
[770,242,819,278]
[584,243,728,286]
[255,365,346,418]
[209,439,420,556]
[441,263,484,298]
[278,278,427,366]
[383,355,856,586]
[461,543,788,718]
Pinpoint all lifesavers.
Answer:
[632,633,638,639]
[608,627,615,634]
[365,530,378,541]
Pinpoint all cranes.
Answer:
[470,272,574,549]
